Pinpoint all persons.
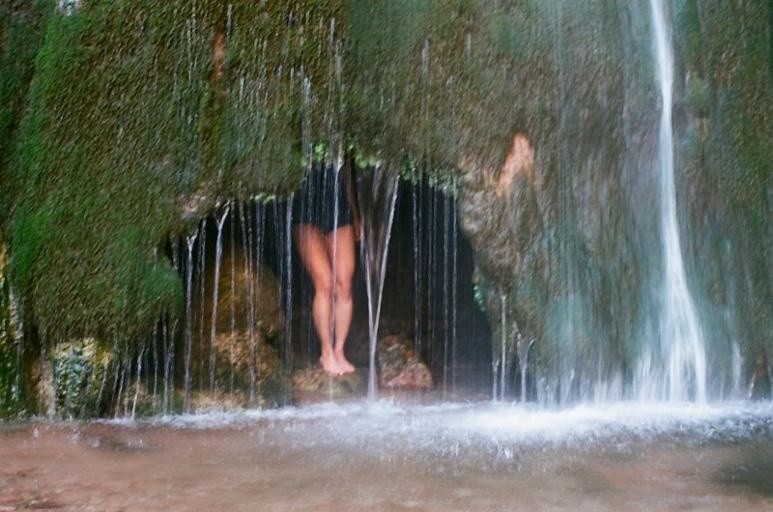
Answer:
[292,160,362,376]
